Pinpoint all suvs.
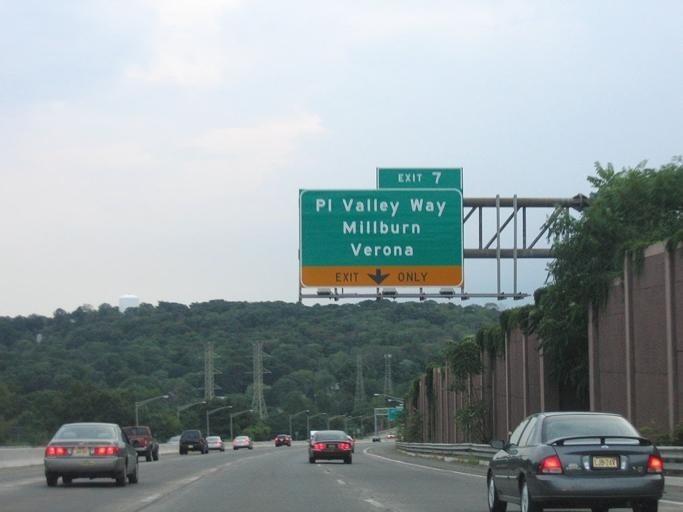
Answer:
[123,425,158,461]
[178,429,208,454]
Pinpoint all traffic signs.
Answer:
[298,189,463,289]
[389,408,403,421]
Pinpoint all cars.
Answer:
[484,410,665,511]
[309,430,396,464]
[205,435,224,452]
[43,421,138,487]
[232,435,253,449]
[274,434,290,446]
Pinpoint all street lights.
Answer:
[134,395,169,425]
[305,412,372,439]
[229,409,253,439]
[373,393,404,403]
[287,409,308,439]
[176,400,207,423]
[206,405,232,434]
